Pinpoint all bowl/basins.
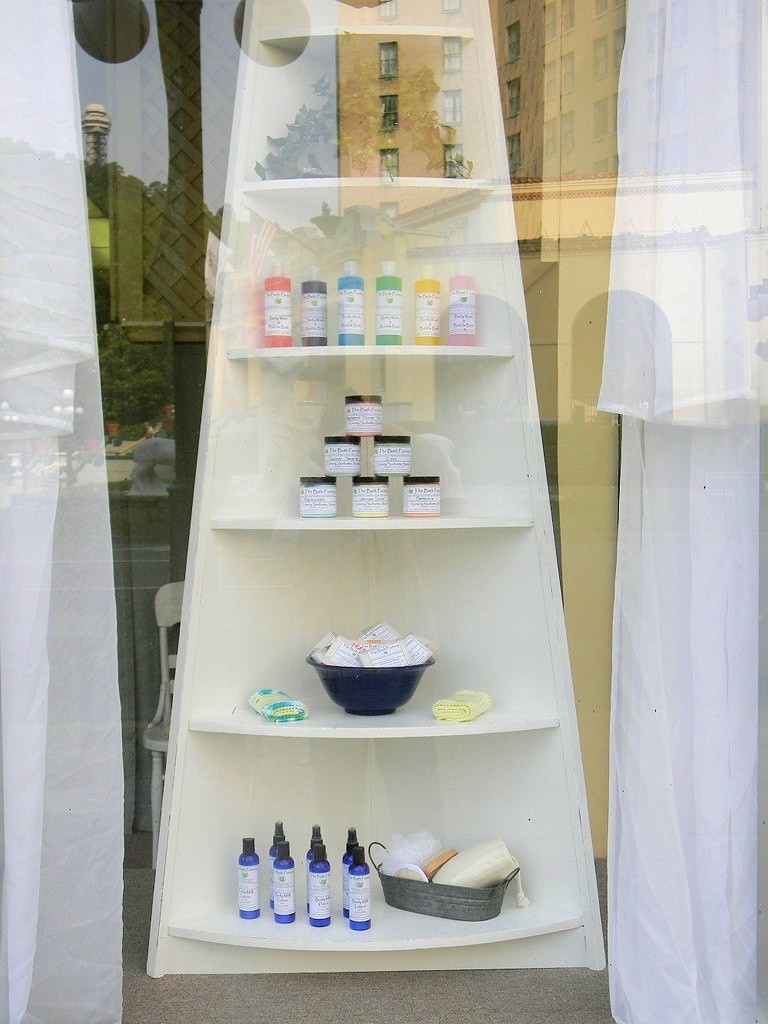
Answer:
[306,655,436,715]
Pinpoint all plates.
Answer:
[391,864,429,884]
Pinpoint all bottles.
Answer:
[300,267,327,346]
[307,825,322,913]
[448,260,476,346]
[375,261,403,345]
[414,264,442,344]
[349,847,371,931]
[342,828,359,917]
[309,844,331,927]
[264,261,292,348]
[337,259,363,346]
[274,842,296,924]
[238,838,260,919]
[270,823,285,909]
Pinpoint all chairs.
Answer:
[142,580,184,871]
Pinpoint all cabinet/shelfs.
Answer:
[147,1,607,980]
